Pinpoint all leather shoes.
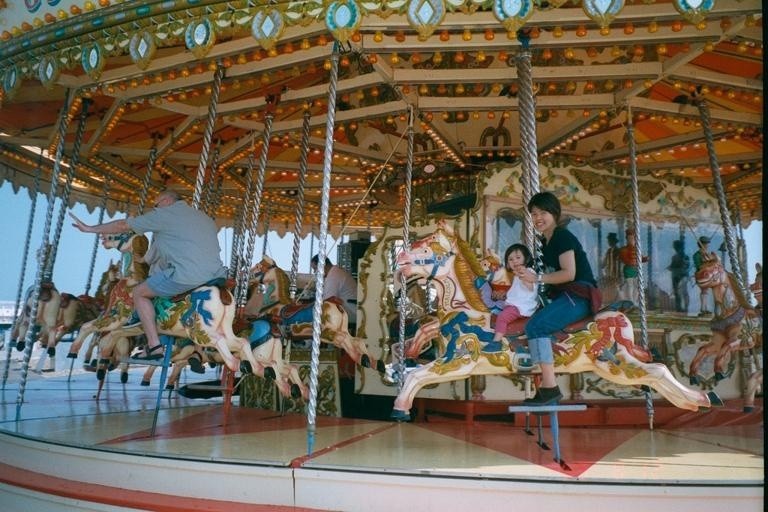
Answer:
[481,341,502,354]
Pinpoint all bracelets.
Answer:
[538,271,544,282]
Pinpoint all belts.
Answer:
[347,299,356,304]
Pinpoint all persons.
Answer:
[667,240,690,312]
[513,192,603,406]
[312,252,357,323]
[602,229,651,302]
[69,189,227,359]
[481,244,541,354]
[693,236,713,315]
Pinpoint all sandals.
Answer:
[131,344,164,360]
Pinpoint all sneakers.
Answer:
[523,385,563,406]
